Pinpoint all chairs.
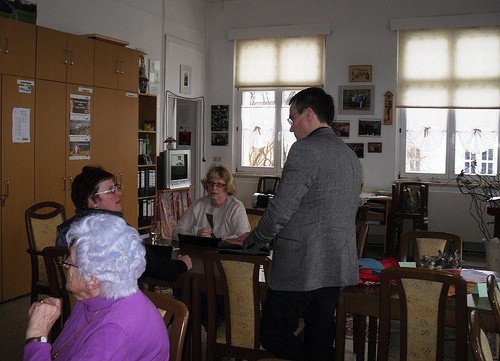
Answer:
[24,200,500,361]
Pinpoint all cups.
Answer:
[151,220,161,242]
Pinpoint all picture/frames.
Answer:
[180,64,192,95]
[331,64,383,158]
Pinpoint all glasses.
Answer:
[59,258,78,270]
[207,182,226,188]
[94,183,122,196]
[287,106,307,126]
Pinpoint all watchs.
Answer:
[25,336,48,345]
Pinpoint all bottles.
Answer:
[138,55,149,93]
[211,105,229,146]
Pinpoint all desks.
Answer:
[362,195,391,258]
[143,235,500,361]
[158,188,193,237]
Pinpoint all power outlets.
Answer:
[212,156,223,162]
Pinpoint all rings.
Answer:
[39,299,45,302]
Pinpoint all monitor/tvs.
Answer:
[158,150,194,189]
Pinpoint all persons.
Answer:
[54,165,252,335]
[23,214,170,361]
[222,88,364,361]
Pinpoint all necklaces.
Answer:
[51,309,102,360]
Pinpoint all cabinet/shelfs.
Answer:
[0,17,158,301]
